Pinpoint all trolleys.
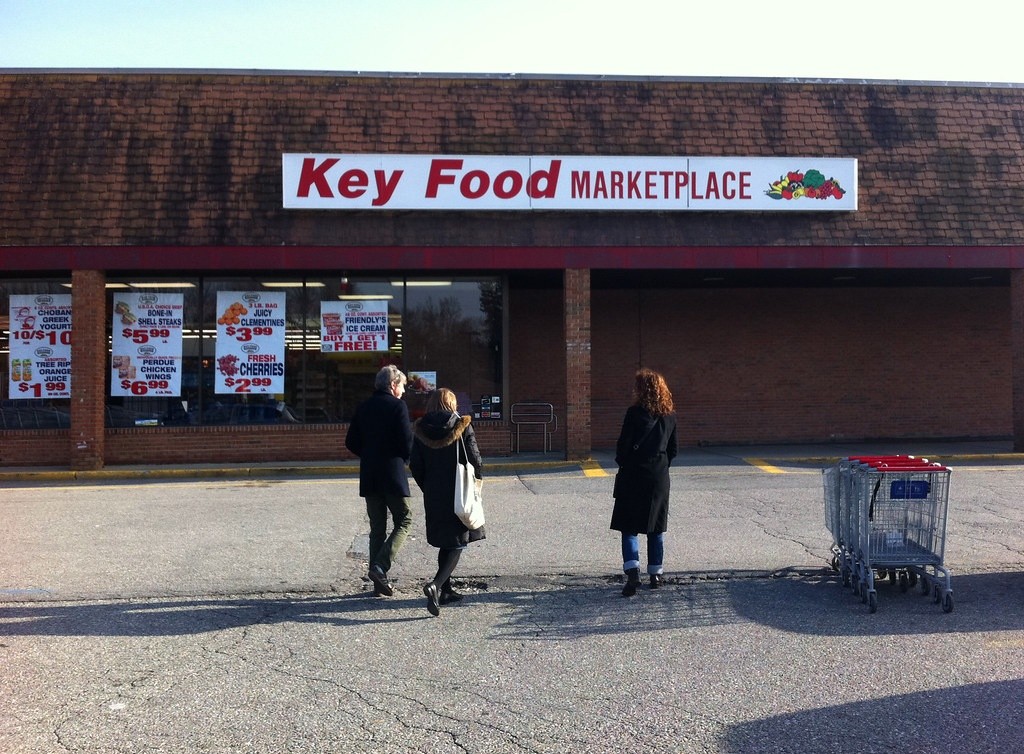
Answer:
[821,454,955,614]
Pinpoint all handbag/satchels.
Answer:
[454,461,485,530]
[613,466,622,498]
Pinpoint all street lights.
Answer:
[469,329,480,398]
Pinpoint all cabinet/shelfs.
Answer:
[295,367,344,422]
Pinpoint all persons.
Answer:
[345,364,413,596]
[47,404,56,411]
[409,387,487,616]
[610,368,679,599]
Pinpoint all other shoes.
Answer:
[650,572,659,588]
[442,591,464,605]
[622,569,641,597]
[368,566,393,597]
[423,581,439,616]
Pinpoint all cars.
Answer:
[402,390,475,421]
[342,390,372,421]
[205,403,302,425]
[182,356,216,389]
